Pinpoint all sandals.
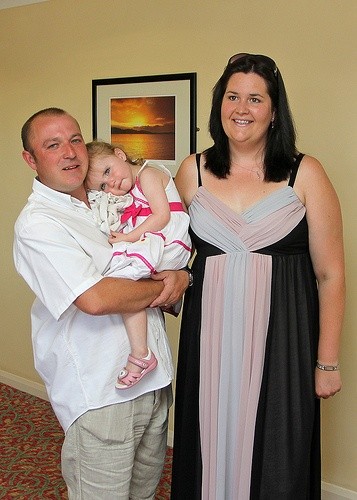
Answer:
[115,347,158,390]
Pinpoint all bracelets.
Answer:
[183,266,194,287]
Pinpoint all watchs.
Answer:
[316,360,342,372]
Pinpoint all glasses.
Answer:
[227,53,279,78]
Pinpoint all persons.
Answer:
[11,108,193,500]
[81,141,191,391]
[172,52,343,500]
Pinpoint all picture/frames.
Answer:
[92,72,197,181]
